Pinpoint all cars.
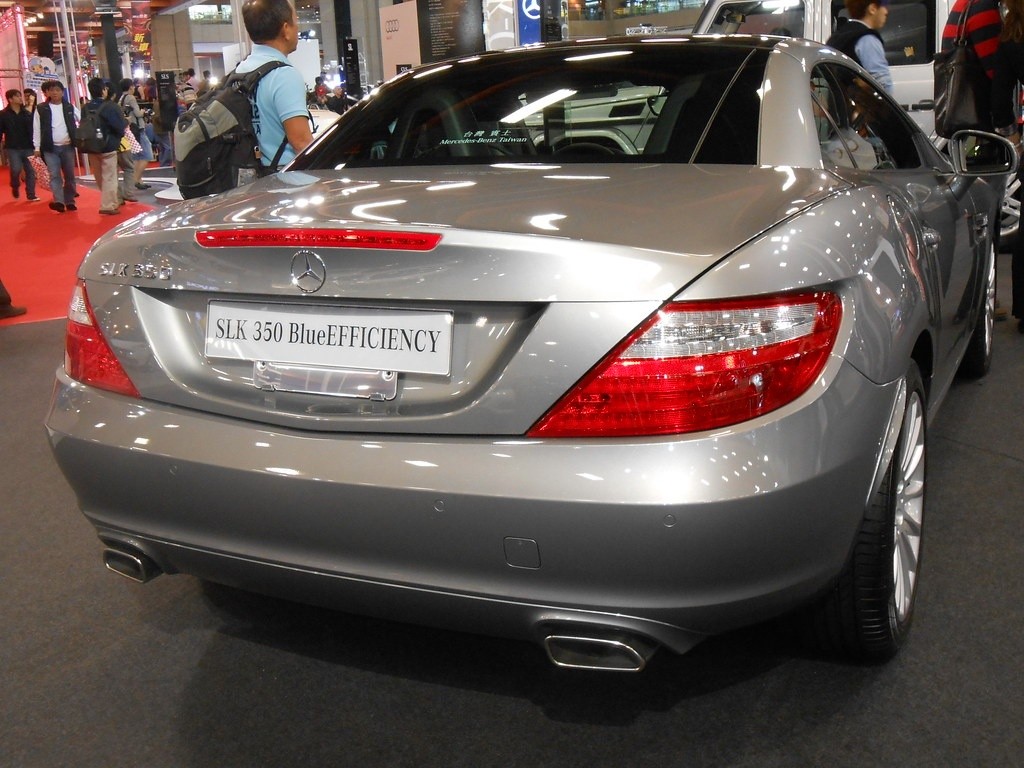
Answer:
[43,33,1016,687]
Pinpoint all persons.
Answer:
[220,0,318,175]
[309,74,349,115]
[0,67,215,215]
[822,1,1023,333]
[0,279,28,321]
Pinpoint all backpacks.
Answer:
[174,61,319,204]
[74,99,111,154]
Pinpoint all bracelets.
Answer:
[1010,140,1023,149]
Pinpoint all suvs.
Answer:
[518,0,1024,253]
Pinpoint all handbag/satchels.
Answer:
[124,127,142,154]
[27,155,52,192]
[932,41,998,139]
[118,137,132,152]
[127,123,141,143]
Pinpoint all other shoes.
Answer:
[66,204,77,210]
[133,182,151,190]
[12,187,19,198]
[994,299,1008,319]
[49,202,64,213]
[0,304,27,319]
[99,209,120,214]
[122,197,138,202]
[27,194,40,201]
